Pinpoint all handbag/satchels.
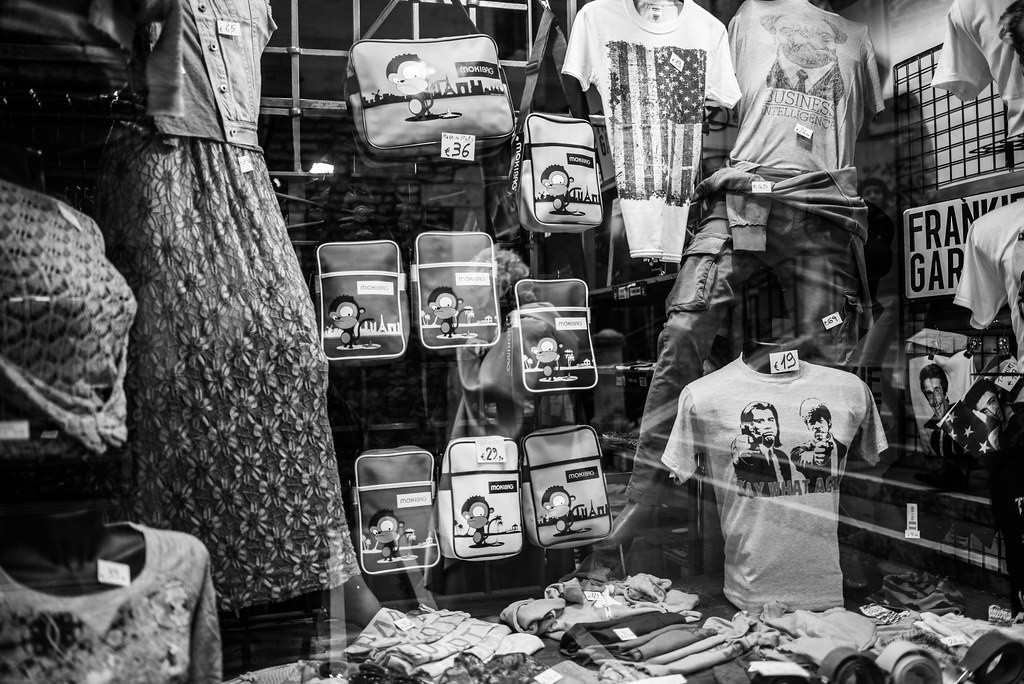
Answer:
[512,113,604,234]
[344,33,517,168]
[351,425,613,575]
[317,232,597,395]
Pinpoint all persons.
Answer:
[594,0,886,553]
[919,364,959,455]
[1,177,157,494]
[963,380,1020,452]
[424,244,581,611]
[662,334,887,613]
[1,473,224,684]
[95,0,387,636]
